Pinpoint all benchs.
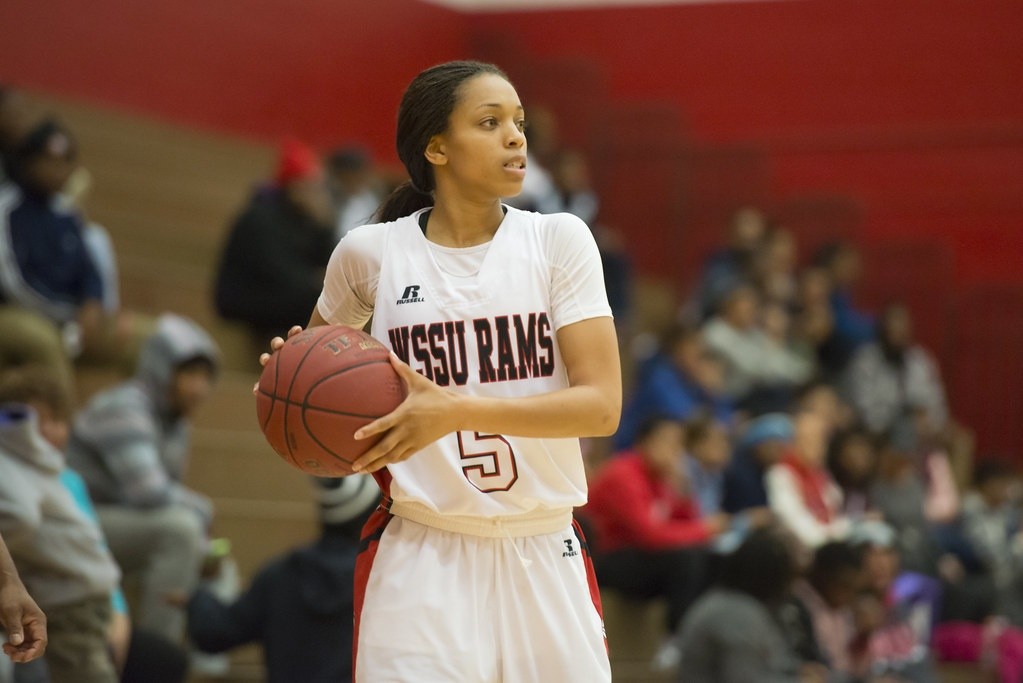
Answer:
[14,91,670,683]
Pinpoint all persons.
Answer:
[0,80,239,682]
[252,61,624,683]
[168,471,384,682]
[500,103,1022,683]
[209,135,398,338]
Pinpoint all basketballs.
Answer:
[257,325,409,478]
